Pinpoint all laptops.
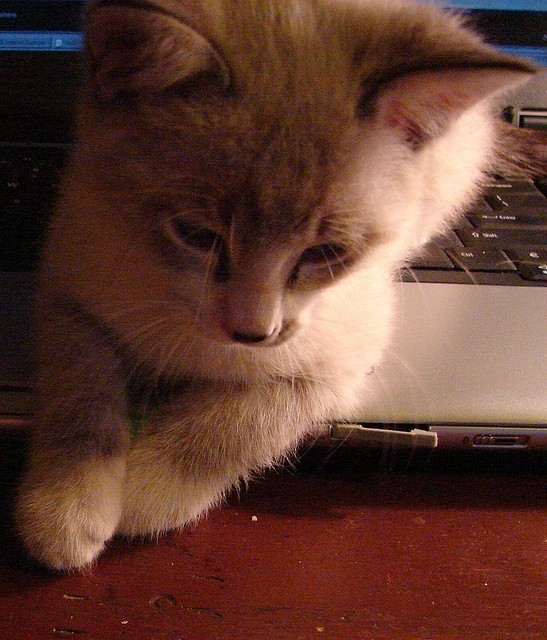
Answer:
[1,2,546,453]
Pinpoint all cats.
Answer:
[13,0,544,575]
